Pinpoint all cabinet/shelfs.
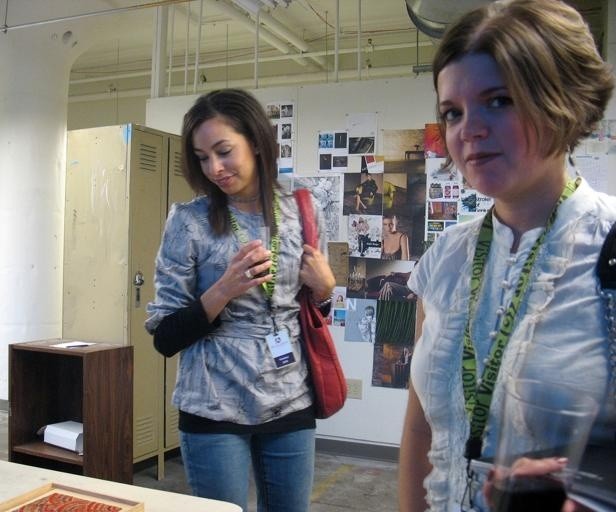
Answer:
[63,126,200,484]
[8,339,134,484]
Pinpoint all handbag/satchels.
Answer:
[299,292,347,419]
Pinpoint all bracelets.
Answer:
[311,294,334,308]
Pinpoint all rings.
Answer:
[244,270,254,279]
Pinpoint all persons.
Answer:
[360,306,376,342]
[354,174,377,211]
[398,0,616,512]
[382,215,408,260]
[144,89,336,512]
[336,295,343,309]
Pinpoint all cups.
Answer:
[487,378,599,511]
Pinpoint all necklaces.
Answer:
[226,195,262,203]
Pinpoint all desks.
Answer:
[0,459,243,512]
